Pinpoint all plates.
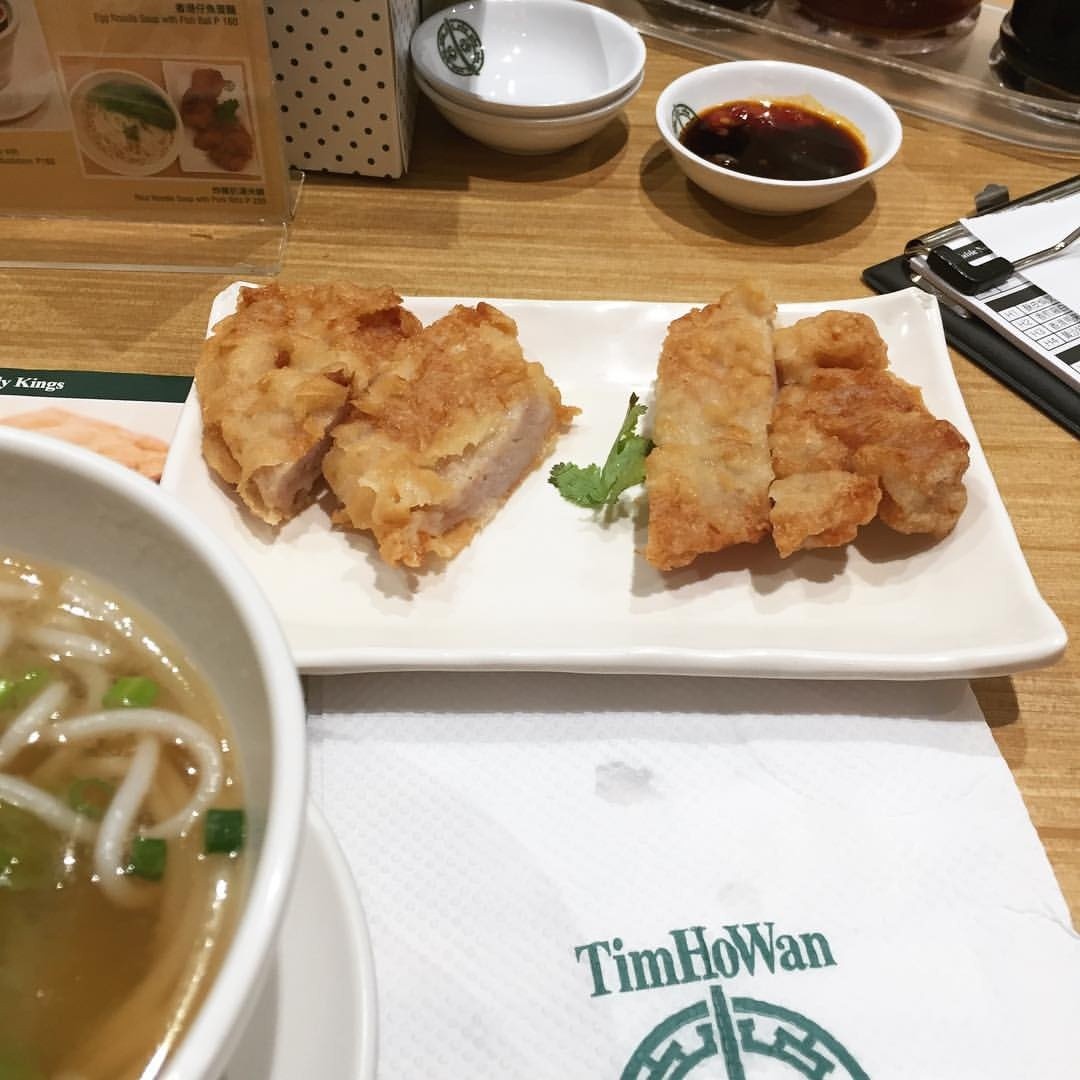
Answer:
[160,282,1069,677]
[0,52,52,123]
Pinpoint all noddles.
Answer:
[2,543,233,1076]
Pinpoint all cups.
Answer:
[0,0,20,91]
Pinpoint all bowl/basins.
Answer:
[68,67,185,176]
[655,59,902,218]
[217,794,380,1076]
[2,425,305,1080]
[409,1,648,155]
